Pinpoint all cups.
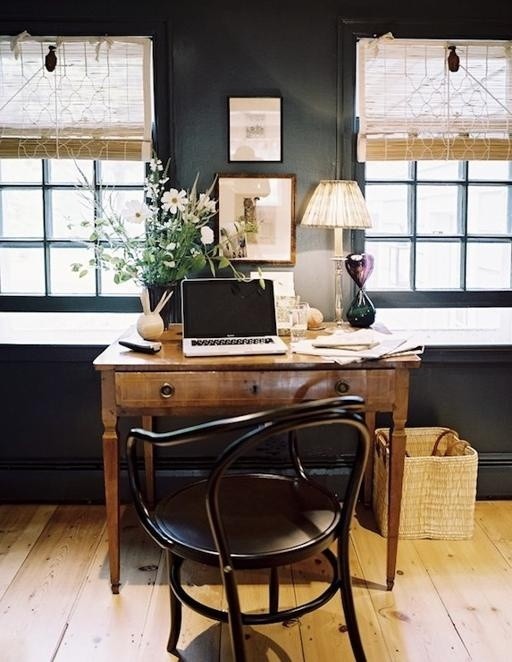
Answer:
[288,302,308,338]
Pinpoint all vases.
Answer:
[144,281,177,332]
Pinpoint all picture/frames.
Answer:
[226,94,285,163]
[213,172,297,267]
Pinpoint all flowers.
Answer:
[63,148,268,291]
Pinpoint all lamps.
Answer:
[298,178,373,335]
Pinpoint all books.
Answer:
[317,335,380,351]
[312,334,374,345]
[379,338,424,358]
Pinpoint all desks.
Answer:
[92,318,424,595]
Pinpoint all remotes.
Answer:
[118,339,162,354]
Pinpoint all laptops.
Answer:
[180,277,289,358]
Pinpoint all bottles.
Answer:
[278,295,290,336]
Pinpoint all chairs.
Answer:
[124,394,372,662]
